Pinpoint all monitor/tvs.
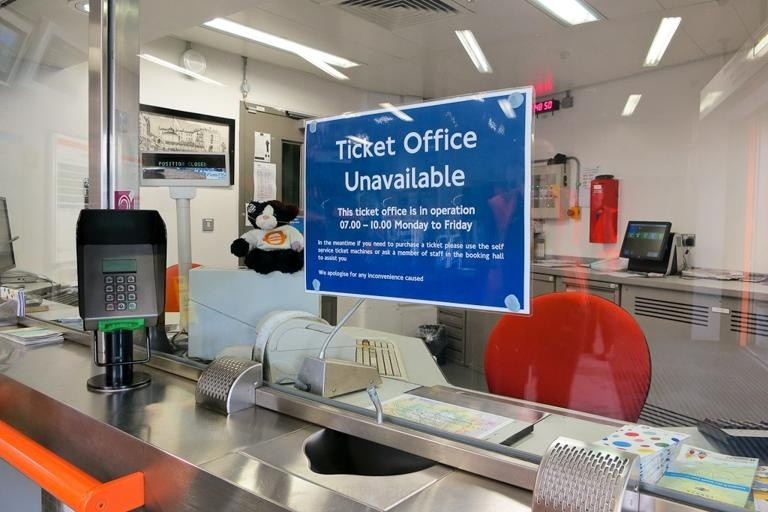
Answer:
[1,6,37,90]
[0,196,16,273]
[619,221,672,262]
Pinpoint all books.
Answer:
[595,424,768,512]
[1,325,64,345]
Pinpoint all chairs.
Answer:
[482,292,653,424]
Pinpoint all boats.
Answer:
[139,115,225,168]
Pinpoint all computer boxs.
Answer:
[187,266,324,362]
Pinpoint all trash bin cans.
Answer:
[418,324,446,365]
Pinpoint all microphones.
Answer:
[365,387,384,424]
[0,234,20,252]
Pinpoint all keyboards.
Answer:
[355,338,408,381]
[41,287,79,307]
[696,418,768,447]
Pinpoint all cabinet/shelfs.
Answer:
[555,277,620,310]
[465,273,555,374]
